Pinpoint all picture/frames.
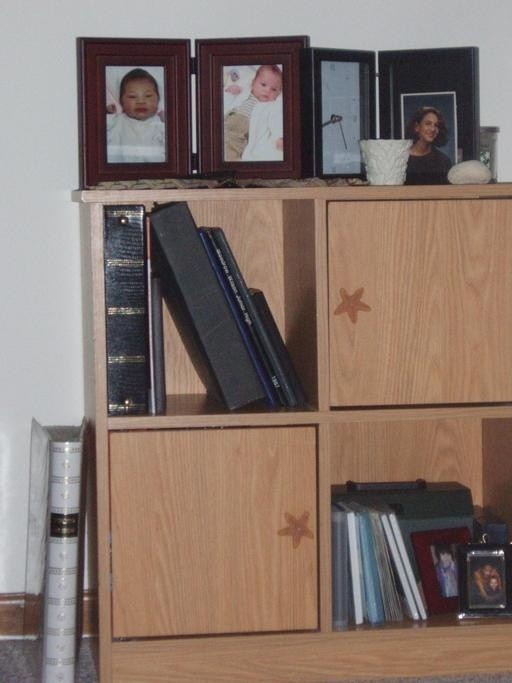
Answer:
[377,47,479,185]
[195,34,309,181]
[76,37,191,190]
[410,528,471,615]
[457,542,512,621]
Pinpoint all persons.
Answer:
[470,558,504,605]
[105,67,167,163]
[221,62,284,161]
[430,544,459,598]
[405,104,454,174]
[483,574,500,597]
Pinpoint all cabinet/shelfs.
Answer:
[72,184,512,683]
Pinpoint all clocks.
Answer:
[312,47,376,181]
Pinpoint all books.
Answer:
[328,490,429,627]
[103,200,306,417]
[22,415,90,681]
